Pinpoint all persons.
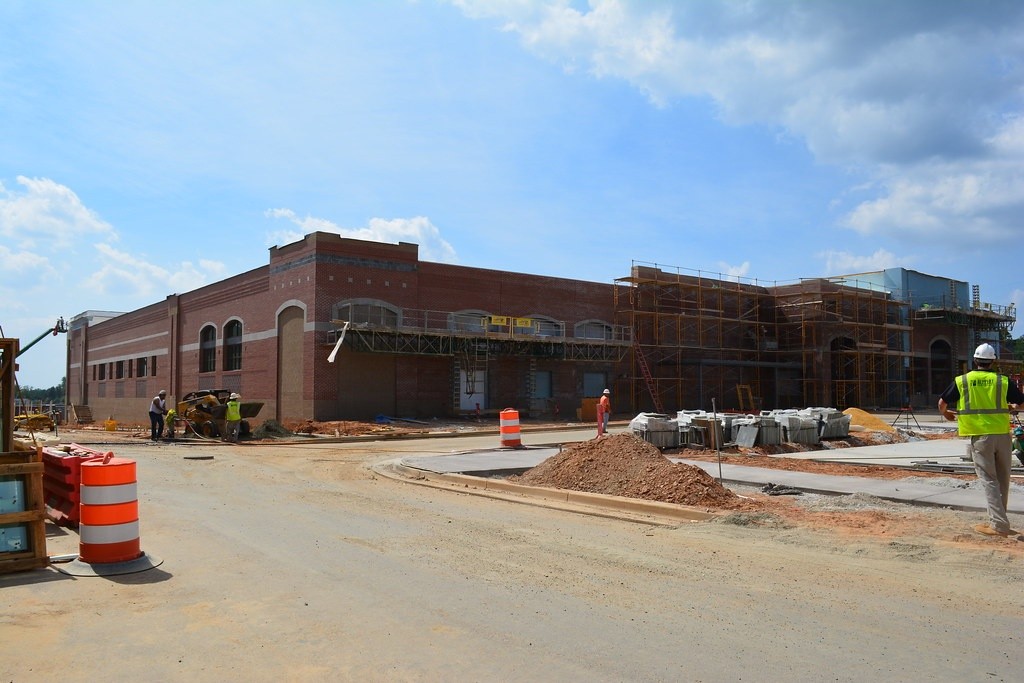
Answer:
[149,390,168,441]
[600,388,611,433]
[1010,427,1024,465]
[937,342,1024,539]
[921,300,929,317]
[224,393,241,443]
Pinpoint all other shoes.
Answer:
[157,435,165,438]
[224,439,233,442]
[231,440,236,443]
[602,430,608,433]
[151,437,157,442]
[974,523,1007,538]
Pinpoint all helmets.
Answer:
[1014,428,1023,436]
[973,343,996,360]
[158,390,166,395]
[603,389,610,394]
[229,393,238,399]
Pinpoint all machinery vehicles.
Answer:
[13,319,68,432]
[177,388,264,438]
